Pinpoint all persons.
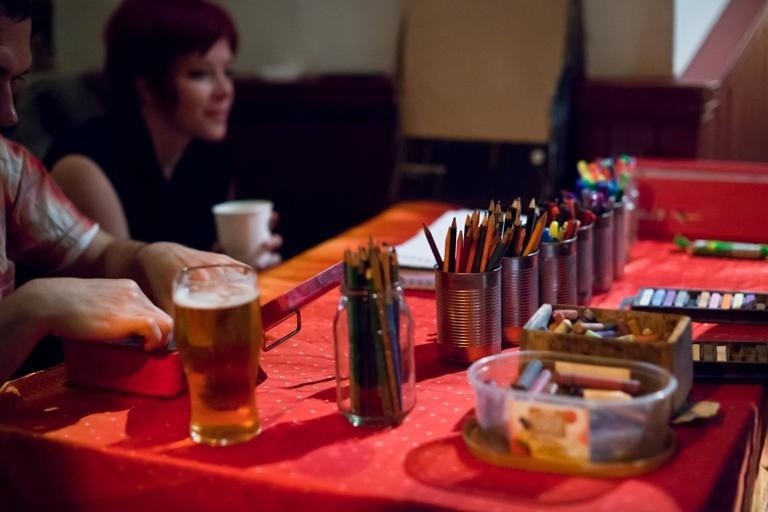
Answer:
[0,0,250,383]
[49,0,284,268]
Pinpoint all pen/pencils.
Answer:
[516,357,552,399]
[575,154,637,214]
[545,191,598,227]
[342,232,403,418]
[541,219,581,242]
[488,196,549,257]
[555,370,643,396]
[422,222,444,272]
[562,188,616,215]
[443,208,516,273]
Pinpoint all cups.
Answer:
[175,203,275,446]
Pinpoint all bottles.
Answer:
[331,287,414,426]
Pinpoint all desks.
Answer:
[1,197,767,511]
